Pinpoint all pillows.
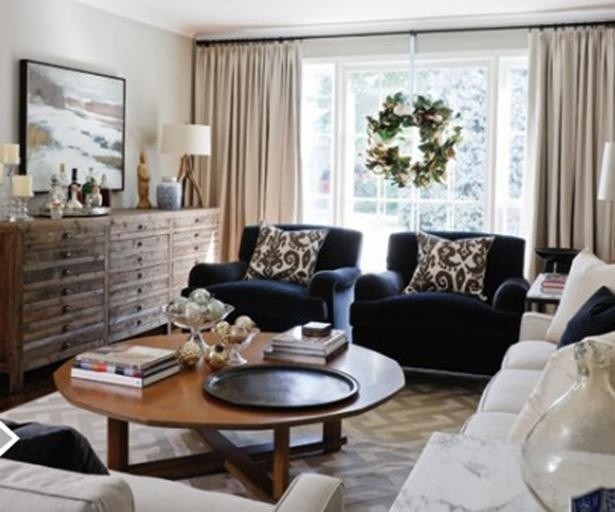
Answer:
[400,230,500,304]
[556,282,614,348]
[544,246,614,344]
[240,220,335,288]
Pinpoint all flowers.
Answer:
[362,90,466,191]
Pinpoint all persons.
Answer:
[136,147,154,211]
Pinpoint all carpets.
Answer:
[1,389,421,512]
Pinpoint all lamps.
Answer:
[596,139,615,264]
[158,122,214,210]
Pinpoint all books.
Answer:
[540,272,569,287]
[260,324,352,366]
[70,342,183,389]
[540,286,564,295]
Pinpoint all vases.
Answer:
[157,175,182,208]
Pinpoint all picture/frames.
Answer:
[14,54,127,195]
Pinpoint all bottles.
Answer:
[519,338,615,511]
[45,161,111,220]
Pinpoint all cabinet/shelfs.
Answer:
[0,213,109,396]
[109,208,172,350]
[169,207,225,307]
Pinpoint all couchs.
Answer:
[348,224,538,383]
[1,412,353,512]
[182,217,366,336]
[385,245,614,510]
